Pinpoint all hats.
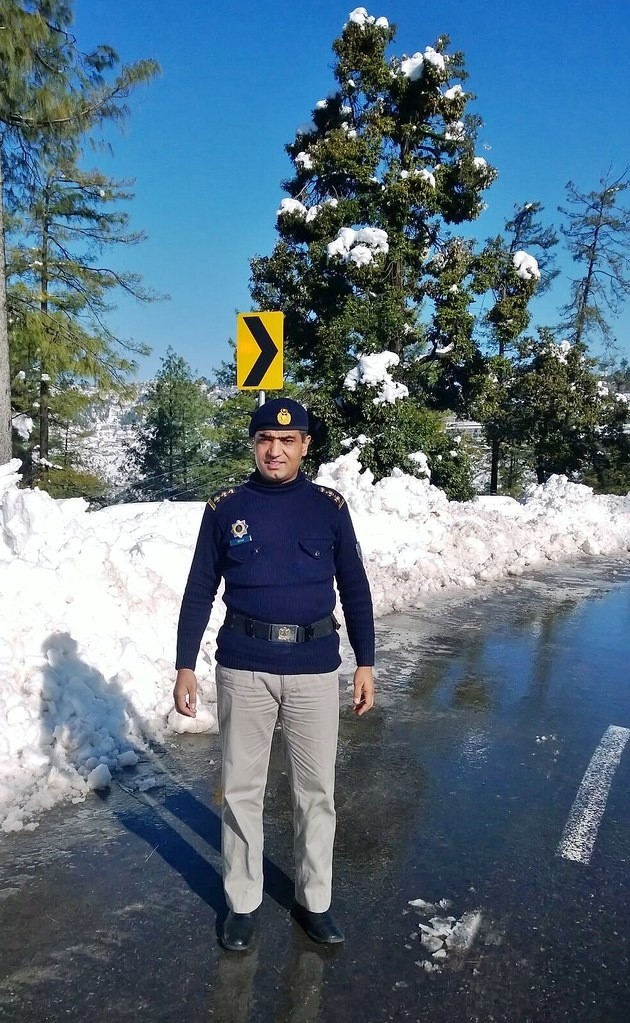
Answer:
[249,398,308,438]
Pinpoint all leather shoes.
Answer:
[295,898,344,944]
[221,904,262,950]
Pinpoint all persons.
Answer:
[175,397,375,951]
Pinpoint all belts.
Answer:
[223,611,342,644]
